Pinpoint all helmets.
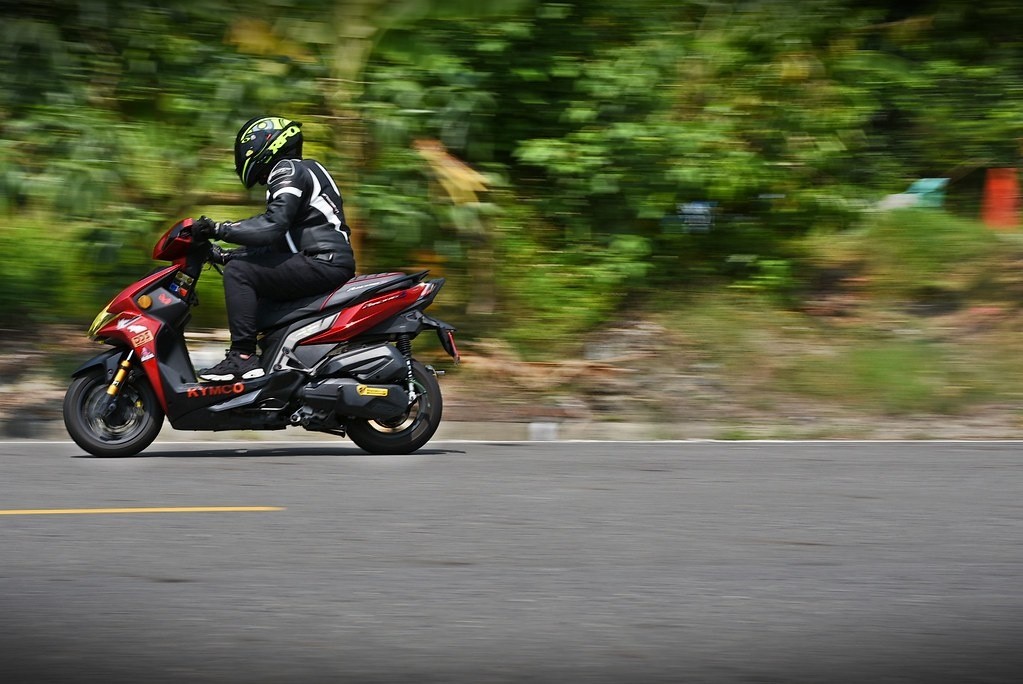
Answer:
[235,116,303,189]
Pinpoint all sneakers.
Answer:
[197,351,265,381]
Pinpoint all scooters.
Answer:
[60,215,459,459]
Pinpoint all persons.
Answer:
[191,116,356,381]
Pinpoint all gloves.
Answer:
[190,215,220,242]
[209,244,232,266]
